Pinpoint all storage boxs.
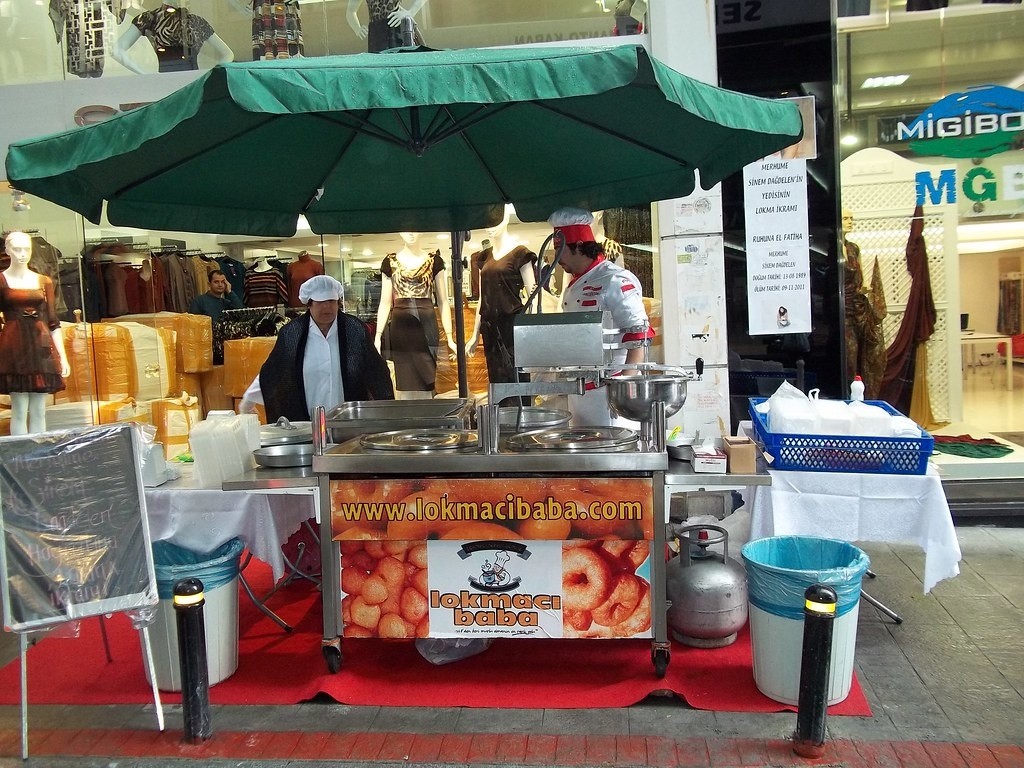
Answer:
[747,396,934,475]
[0,309,279,460]
[688,447,728,474]
[722,436,775,475]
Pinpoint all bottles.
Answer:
[850,375,866,401]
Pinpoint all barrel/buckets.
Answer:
[473,403,574,432]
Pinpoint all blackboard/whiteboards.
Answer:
[0,420,160,633]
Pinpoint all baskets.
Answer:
[748,396,934,475]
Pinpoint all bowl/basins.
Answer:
[603,374,688,422]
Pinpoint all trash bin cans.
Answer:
[138,538,245,694]
[741,535,871,707]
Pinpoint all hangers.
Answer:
[123,243,226,260]
[0,229,62,257]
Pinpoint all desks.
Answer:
[733,420,962,625]
[137,463,324,630]
[961,334,1013,393]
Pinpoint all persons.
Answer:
[241,257,288,310]
[188,270,242,320]
[0,231,70,435]
[558,209,625,314]
[48,0,431,77]
[238,275,396,425]
[840,207,885,400]
[373,232,458,399]
[548,206,654,431]
[285,250,324,307]
[465,205,539,413]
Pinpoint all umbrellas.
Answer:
[3,42,804,402]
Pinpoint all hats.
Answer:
[548,207,595,248]
[299,276,343,304]
